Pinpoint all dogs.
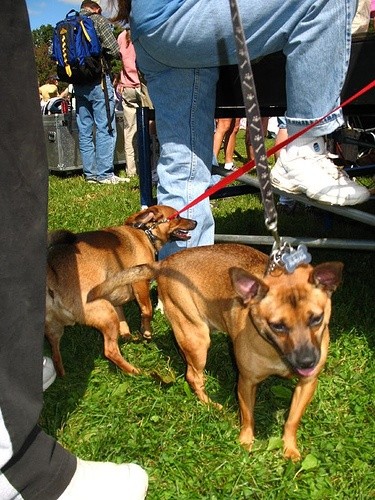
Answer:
[44,205,191,383]
[87,241,345,465]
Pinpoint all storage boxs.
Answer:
[42,111,126,172]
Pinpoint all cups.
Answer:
[61,101,68,114]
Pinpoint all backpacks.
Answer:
[53,10,105,85]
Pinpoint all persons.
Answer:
[0,0,149,500]
[40,0,375,315]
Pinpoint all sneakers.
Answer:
[269,137,370,206]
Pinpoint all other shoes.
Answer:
[88,179,96,183]
[98,175,130,185]
[224,162,239,172]
[212,158,220,167]
